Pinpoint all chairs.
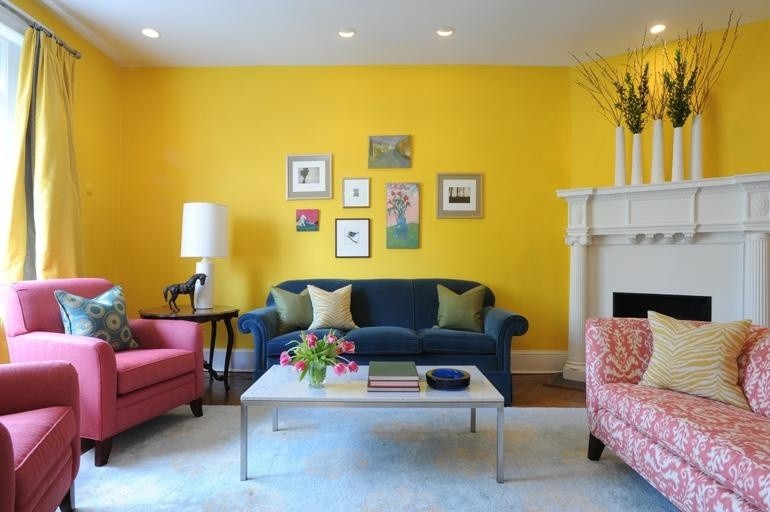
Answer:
[3,278,208,467]
[0,361,82,512]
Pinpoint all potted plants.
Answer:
[660,49,699,183]
[613,62,650,186]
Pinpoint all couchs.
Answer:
[583,314,769,512]
[238,278,530,405]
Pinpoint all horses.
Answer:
[163,273,206,313]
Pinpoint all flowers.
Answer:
[277,329,359,387]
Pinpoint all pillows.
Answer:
[431,284,486,333]
[306,283,360,330]
[638,309,756,414]
[269,285,315,336]
[53,285,140,353]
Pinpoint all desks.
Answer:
[137,304,240,391]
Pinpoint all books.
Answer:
[366,361,420,392]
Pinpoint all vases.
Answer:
[308,360,326,387]
[689,113,705,181]
[651,119,665,185]
[614,125,628,187]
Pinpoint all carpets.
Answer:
[54,401,687,512]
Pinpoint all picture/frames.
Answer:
[341,177,371,208]
[435,172,484,220]
[335,218,371,258]
[285,155,334,201]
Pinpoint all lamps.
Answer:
[178,202,231,310]
